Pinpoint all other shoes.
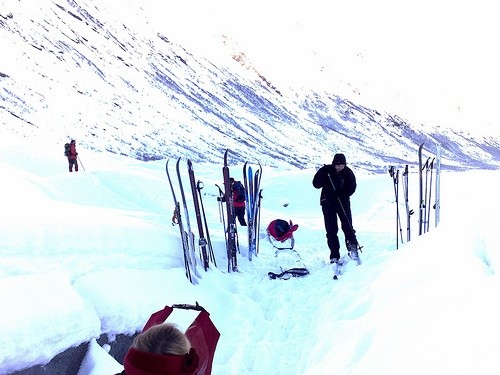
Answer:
[351,251,359,261]
[330,258,338,263]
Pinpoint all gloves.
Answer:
[217,197,221,201]
[336,192,345,200]
[325,165,333,172]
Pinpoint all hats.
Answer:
[332,154,346,166]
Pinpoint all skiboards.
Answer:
[163,156,210,286]
[214,148,264,274]
[388,142,441,249]
[333,249,361,281]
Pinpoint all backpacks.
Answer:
[64,142,71,156]
[233,182,245,202]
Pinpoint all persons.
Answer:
[217,178,247,226]
[111,301,221,375]
[68,140,78,172]
[312,152,359,264]
